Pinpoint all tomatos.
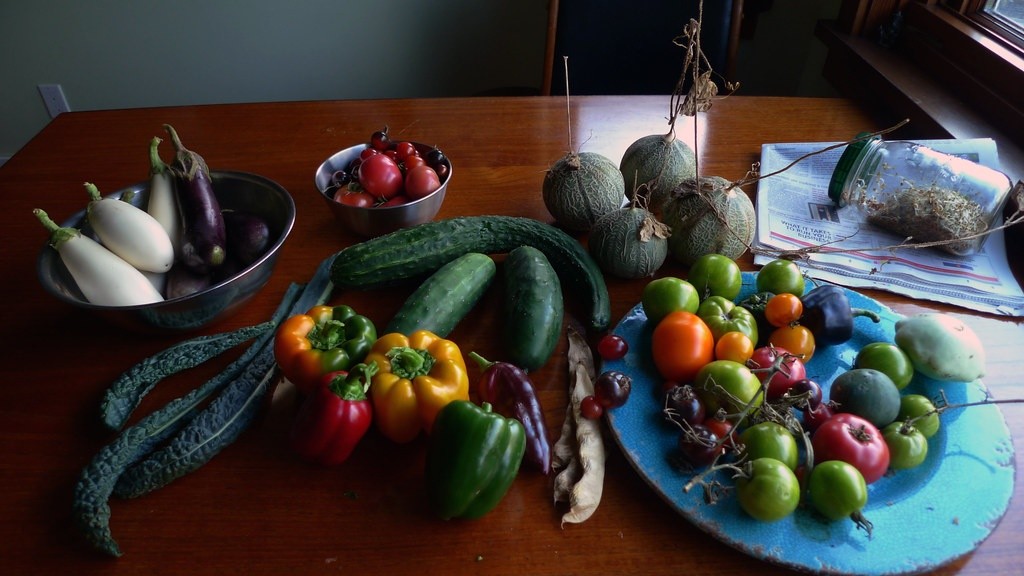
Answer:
[578,335,629,418]
[325,125,448,208]
[640,253,942,521]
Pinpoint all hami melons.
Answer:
[542,19,910,279]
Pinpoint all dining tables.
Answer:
[0,95,1023,576]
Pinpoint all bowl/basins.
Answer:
[39,168,296,340]
[315,141,452,238]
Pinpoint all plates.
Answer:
[597,270,1015,575]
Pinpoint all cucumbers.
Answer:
[329,215,612,330]
[501,245,563,375]
[381,252,499,337]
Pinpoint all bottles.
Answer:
[826,132,1014,258]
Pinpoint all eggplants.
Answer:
[31,123,271,305]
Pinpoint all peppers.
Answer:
[363,330,471,444]
[470,350,551,475]
[293,363,380,464]
[275,304,376,395]
[426,399,526,520]
